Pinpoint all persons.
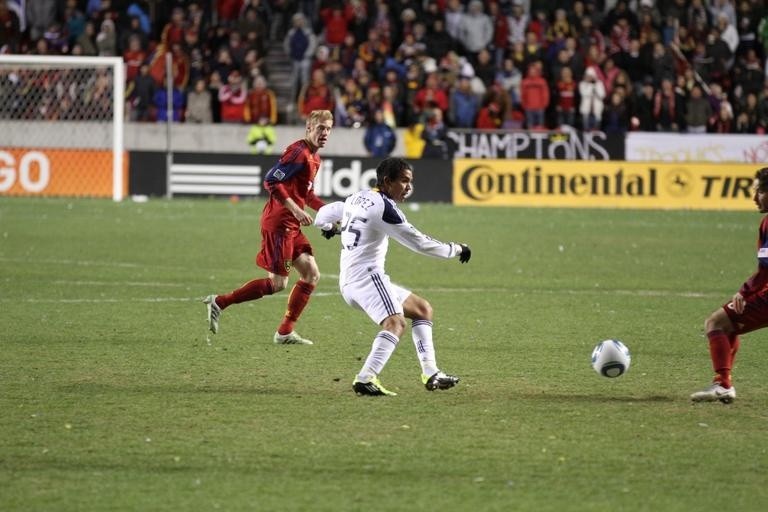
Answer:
[691,167,768,401]
[314,155,471,396]
[248,116,276,156]
[204,109,332,345]
[0,0,768,135]
[403,107,460,160]
[364,109,396,158]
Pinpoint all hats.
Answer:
[586,66,598,80]
[462,63,475,78]
[424,58,438,73]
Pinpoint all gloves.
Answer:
[459,243,471,264]
[321,224,341,239]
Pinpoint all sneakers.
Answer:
[421,369,461,390]
[352,374,397,396]
[206,294,223,334]
[274,330,314,345]
[691,383,736,404]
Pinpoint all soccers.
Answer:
[590,340,631,377]
